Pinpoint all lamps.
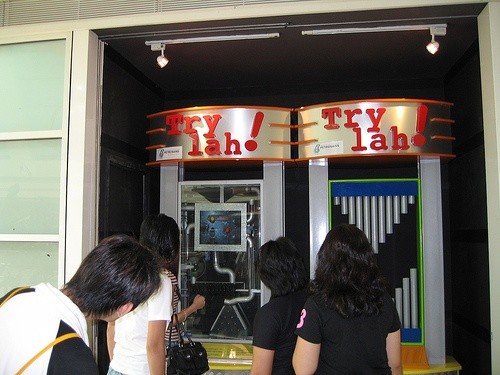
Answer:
[150,43,169,68]
[426,23,447,54]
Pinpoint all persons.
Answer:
[292,224,403,375]
[0,213,206,375]
[250,236,309,375]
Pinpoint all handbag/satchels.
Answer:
[167,313,209,375]
